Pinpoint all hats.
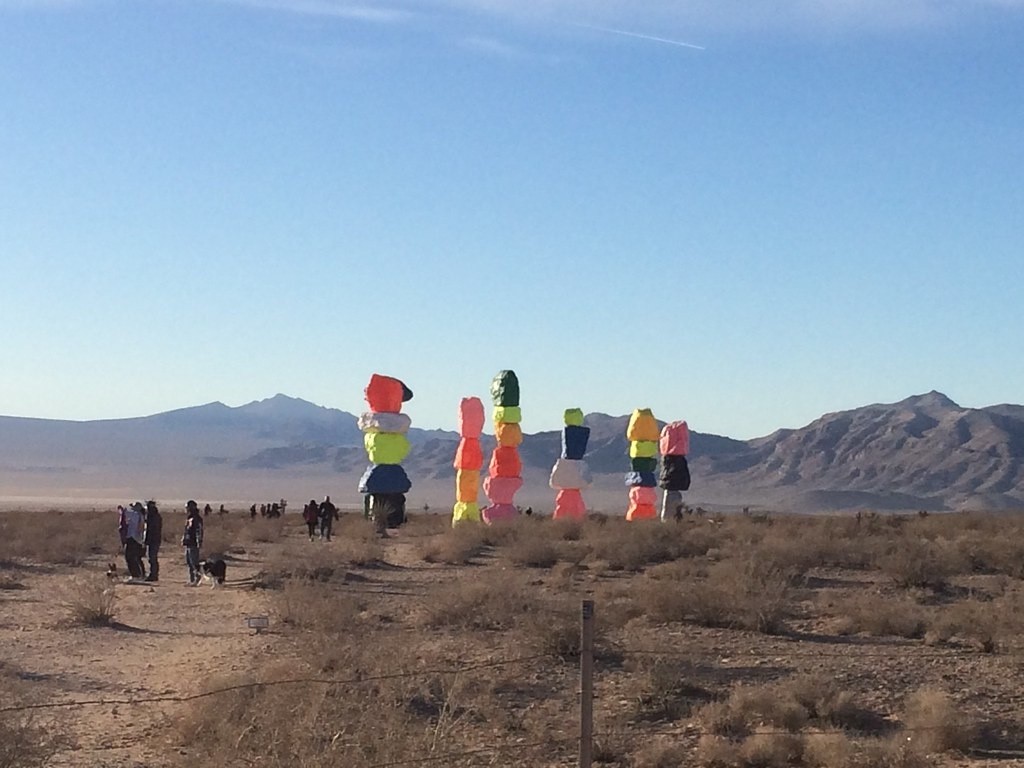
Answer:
[129,501,142,510]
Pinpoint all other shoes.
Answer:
[309,538,314,542]
[194,571,204,586]
[144,573,158,581]
[319,536,323,541]
[126,576,145,584]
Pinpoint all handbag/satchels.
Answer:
[320,507,326,517]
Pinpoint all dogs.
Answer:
[194,559,227,591]
[102,563,134,599]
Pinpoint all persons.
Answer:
[526,507,532,515]
[250,503,282,519]
[303,496,339,542]
[181,500,204,586]
[204,504,228,515]
[119,500,163,581]
